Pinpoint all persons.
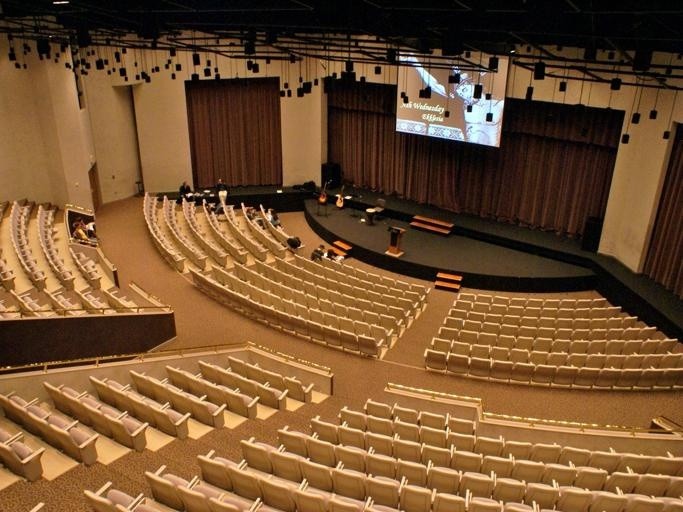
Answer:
[400,52,505,147]
[324,249,345,263]
[214,178,226,190]
[179,181,191,193]
[72,216,96,248]
[246,206,285,230]
[205,202,216,215]
[314,244,325,257]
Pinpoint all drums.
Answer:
[366,208,376,226]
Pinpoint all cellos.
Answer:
[319,182,328,203]
[336,185,344,207]
